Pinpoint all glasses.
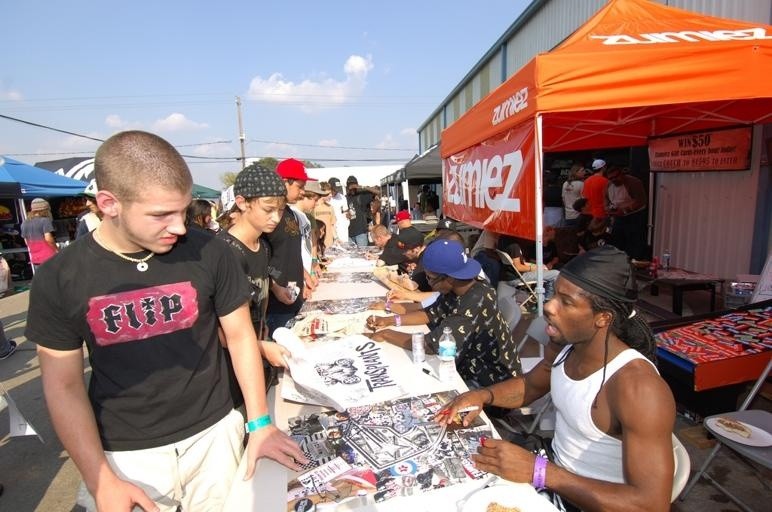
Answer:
[424,274,448,290]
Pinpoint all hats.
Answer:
[230,163,288,212]
[30,198,50,212]
[392,209,412,225]
[303,180,330,197]
[592,159,606,171]
[421,238,482,280]
[83,178,98,197]
[398,226,425,251]
[436,219,457,232]
[346,176,358,185]
[558,243,640,304]
[274,158,319,182]
[328,177,343,195]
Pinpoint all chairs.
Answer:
[491,315,554,452]
[497,296,522,333]
[679,358,772,511]
[475,247,548,308]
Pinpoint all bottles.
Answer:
[438,326,456,383]
[662,248,670,273]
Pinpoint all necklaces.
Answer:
[96,224,154,272]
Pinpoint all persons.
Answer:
[422,203,436,221]
[0,248,18,361]
[21,198,60,279]
[412,202,422,220]
[581,160,609,226]
[570,197,593,245]
[371,224,408,267]
[313,219,326,266]
[562,165,585,224]
[289,181,330,278]
[217,186,247,232]
[75,179,104,236]
[184,198,216,234]
[526,226,561,271]
[391,210,425,248]
[366,197,386,246]
[420,185,431,213]
[23,132,309,511]
[211,164,291,447]
[470,230,513,289]
[417,190,422,203]
[328,178,351,243]
[361,240,523,420]
[431,247,677,512]
[312,182,342,249]
[374,226,429,291]
[386,231,490,309]
[346,176,380,246]
[603,166,650,258]
[264,158,318,325]
[500,234,546,272]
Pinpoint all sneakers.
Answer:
[0,341,17,360]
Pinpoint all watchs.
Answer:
[621,208,627,215]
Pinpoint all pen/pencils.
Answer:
[372,314,376,334]
[386,288,394,303]
[440,405,479,414]
[366,249,369,257]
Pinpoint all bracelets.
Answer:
[307,272,315,276]
[532,454,547,488]
[394,315,401,327]
[244,414,272,433]
[385,301,393,313]
[483,387,494,405]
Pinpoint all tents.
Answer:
[0,152,94,197]
[439,1,772,356]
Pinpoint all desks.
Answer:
[219,244,561,512]
[635,265,725,316]
